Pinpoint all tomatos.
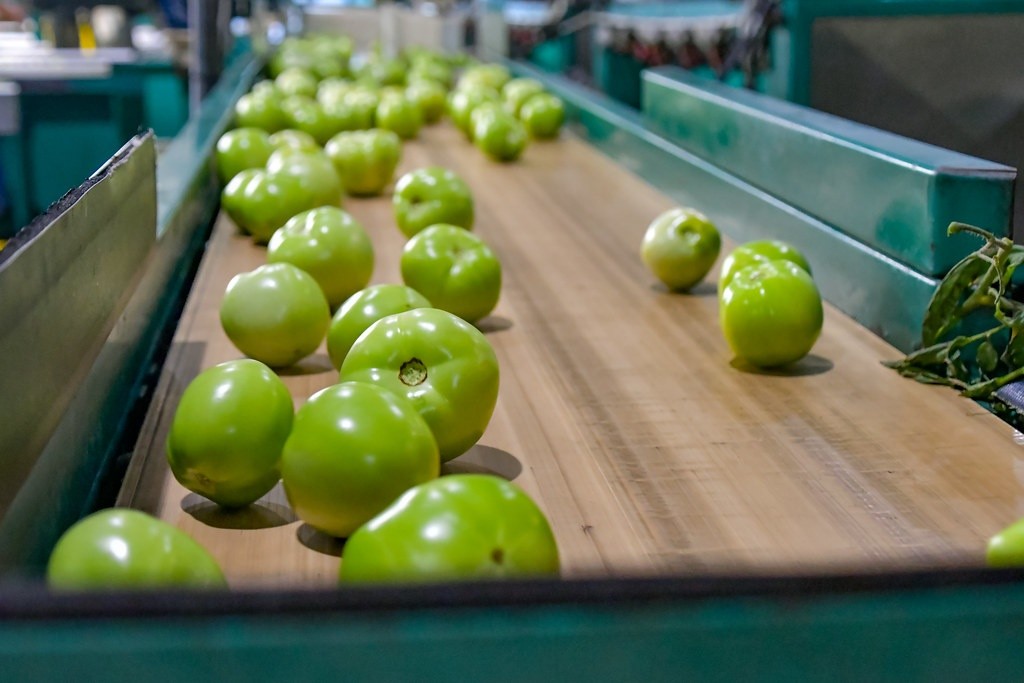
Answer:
[985,519,1024,567]
[45,36,823,589]
